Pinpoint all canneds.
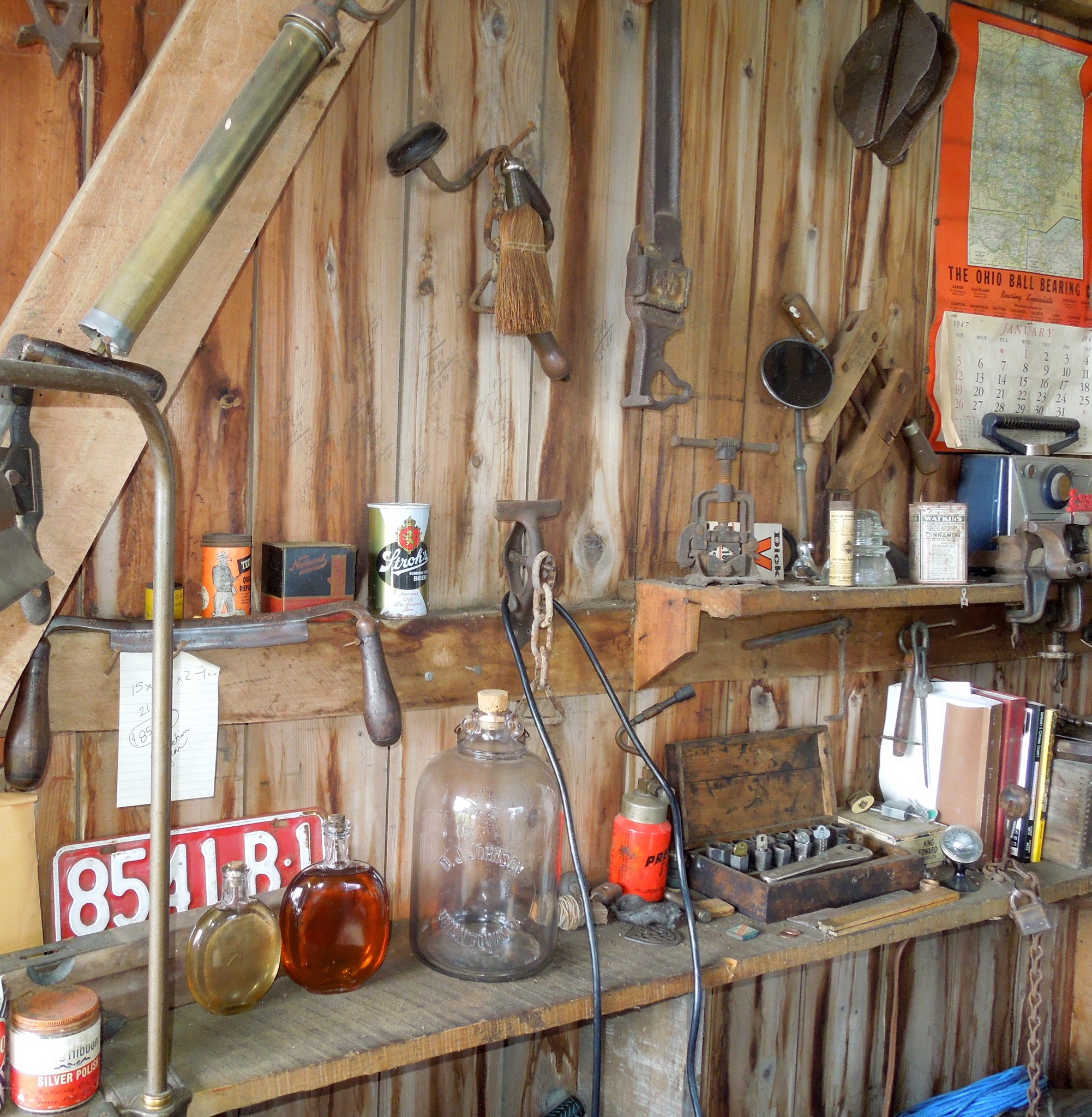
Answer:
[200,532,254,619]
[144,582,183,622]
[366,500,433,619]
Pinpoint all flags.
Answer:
[237,554,251,574]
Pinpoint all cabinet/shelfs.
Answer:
[6,585,1092,1115]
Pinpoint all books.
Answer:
[878,676,1092,873]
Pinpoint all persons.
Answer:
[212,551,238,616]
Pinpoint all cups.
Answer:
[367,501,433,621]
[902,419,939,477]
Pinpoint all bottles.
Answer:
[406,690,561,982]
[187,860,283,1016]
[280,816,395,996]
